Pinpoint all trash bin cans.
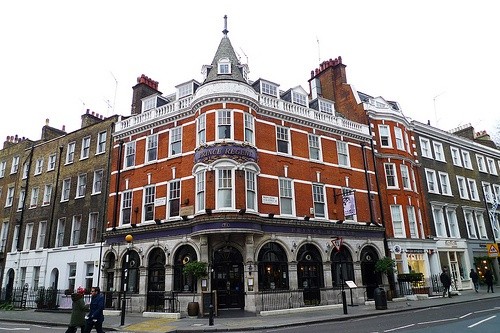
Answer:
[373,286,388,310]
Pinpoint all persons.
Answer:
[342,197,351,211]
[86,287,105,333]
[440,269,451,297]
[469,269,478,293]
[485,271,494,293]
[66,286,89,333]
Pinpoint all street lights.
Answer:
[119,234,133,326]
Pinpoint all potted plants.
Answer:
[374,256,395,301]
[183,260,209,316]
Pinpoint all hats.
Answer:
[77,288,85,293]
[444,268,448,271]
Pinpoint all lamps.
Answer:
[154,219,161,224]
[269,213,274,218]
[206,208,212,216]
[291,240,361,255]
[164,247,170,257]
[131,223,136,228]
[181,215,188,220]
[338,219,343,224]
[138,249,144,258]
[305,216,310,221]
[111,226,116,231]
[238,209,246,215]
[367,221,371,226]
